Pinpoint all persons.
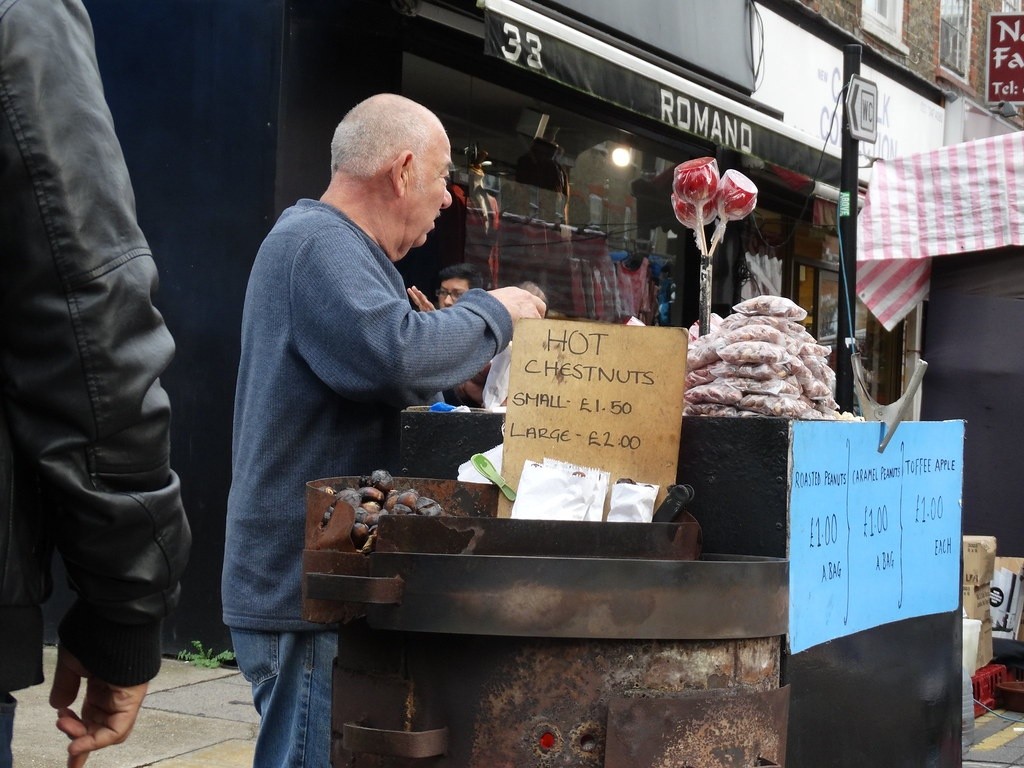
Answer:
[219,93,548,768]
[430,260,547,407]
[0,0,191,768]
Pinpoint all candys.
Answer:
[671,157,759,258]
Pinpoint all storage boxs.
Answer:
[962,534,1024,719]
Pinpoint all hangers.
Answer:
[613,239,654,268]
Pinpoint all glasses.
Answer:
[435,288,467,302]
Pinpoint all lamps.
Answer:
[516,137,566,194]
